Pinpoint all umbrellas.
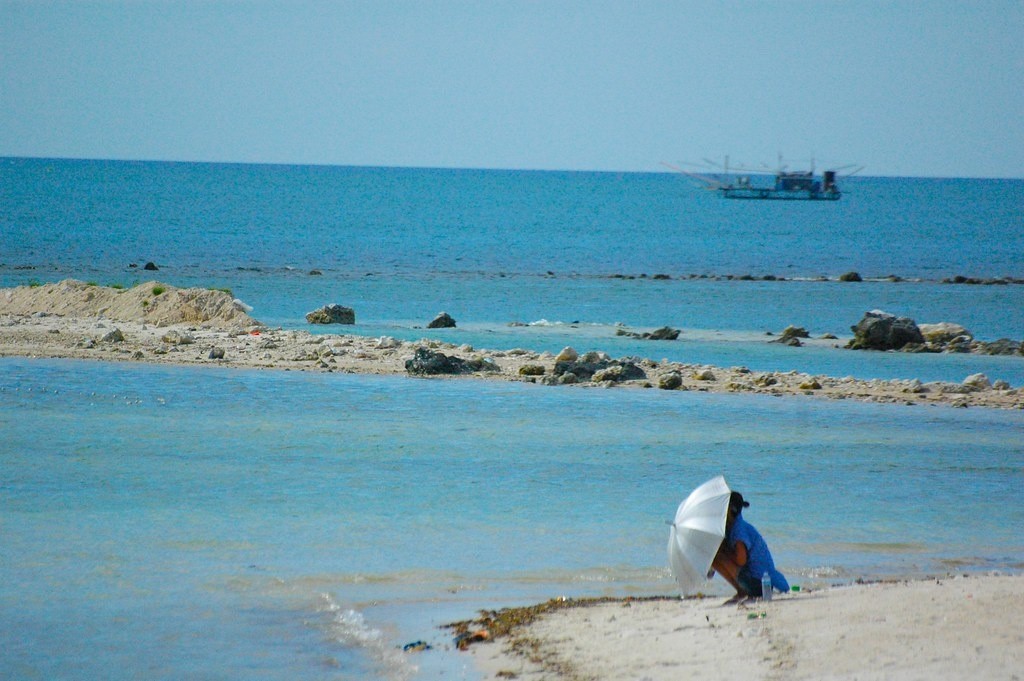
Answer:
[665,474,732,594]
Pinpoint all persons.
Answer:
[713,492,790,606]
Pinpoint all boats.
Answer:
[661,152,863,199]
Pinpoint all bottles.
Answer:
[762,571,771,601]
